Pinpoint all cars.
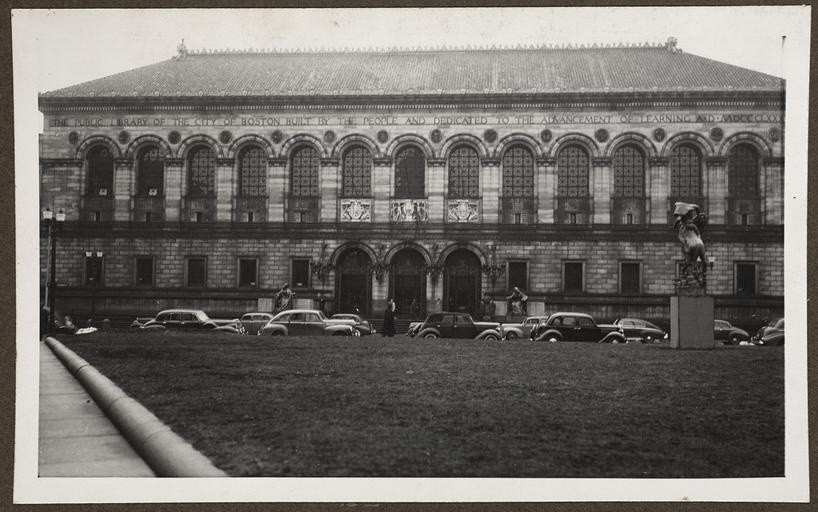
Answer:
[241,311,276,335]
[407,311,503,341]
[532,311,628,344]
[501,315,548,340]
[140,308,246,335]
[260,309,358,336]
[714,319,751,346]
[614,318,666,344]
[331,313,377,337]
[752,317,784,346]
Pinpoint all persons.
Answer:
[447,296,454,311]
[319,296,324,311]
[511,295,523,317]
[275,282,297,312]
[351,295,360,315]
[389,298,395,319]
[506,287,529,317]
[384,302,394,336]
[478,292,496,322]
[435,298,441,313]
[410,297,419,322]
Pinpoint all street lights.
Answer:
[41,207,67,332]
